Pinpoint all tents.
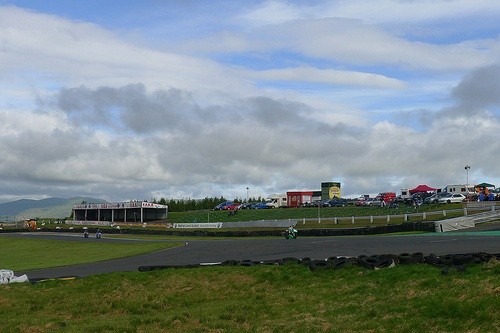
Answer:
[474,183,495,194]
[409,185,437,194]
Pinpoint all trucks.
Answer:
[266,194,288,208]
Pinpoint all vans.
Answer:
[355,192,397,207]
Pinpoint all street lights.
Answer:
[465,164,471,203]
[246,187,249,204]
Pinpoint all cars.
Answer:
[395,189,467,204]
[214,201,270,210]
[304,199,347,207]
[469,187,500,200]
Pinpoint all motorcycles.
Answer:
[282,228,298,239]
[95,232,102,239]
[83,233,89,238]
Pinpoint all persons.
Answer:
[289,226,294,233]
[185,241,189,248]
